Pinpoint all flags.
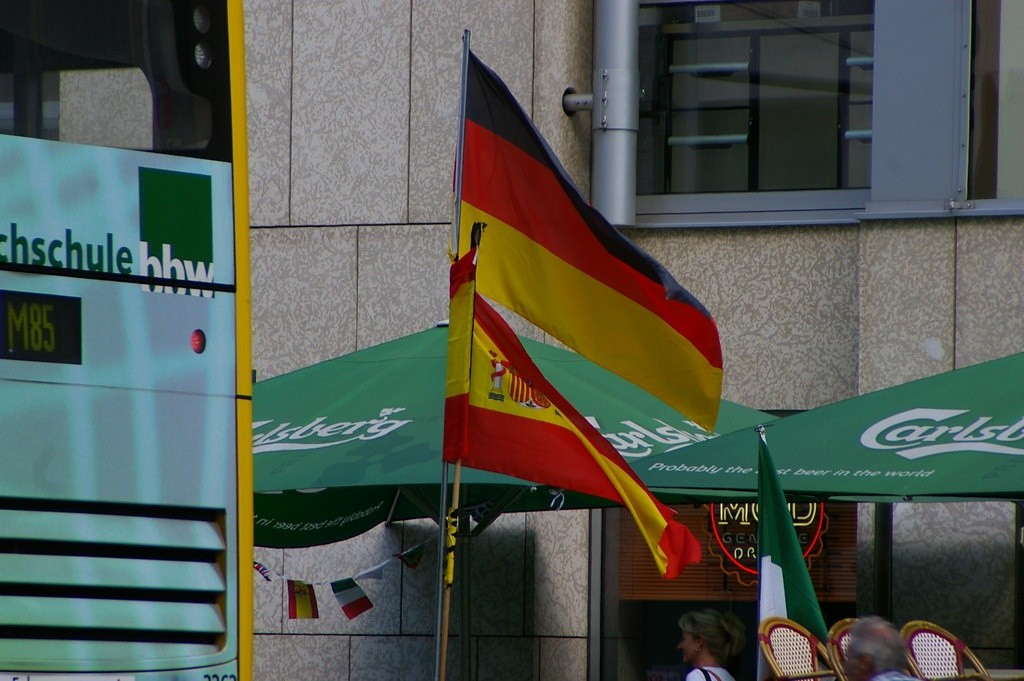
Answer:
[443,252,701,579]
[252,496,499,620]
[757,435,837,681]
[449,50,722,434]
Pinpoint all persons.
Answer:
[846,617,922,681]
[679,610,735,681]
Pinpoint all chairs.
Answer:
[758,616,994,681]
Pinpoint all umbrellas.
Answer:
[251,321,778,681]
[630,351,1024,504]
